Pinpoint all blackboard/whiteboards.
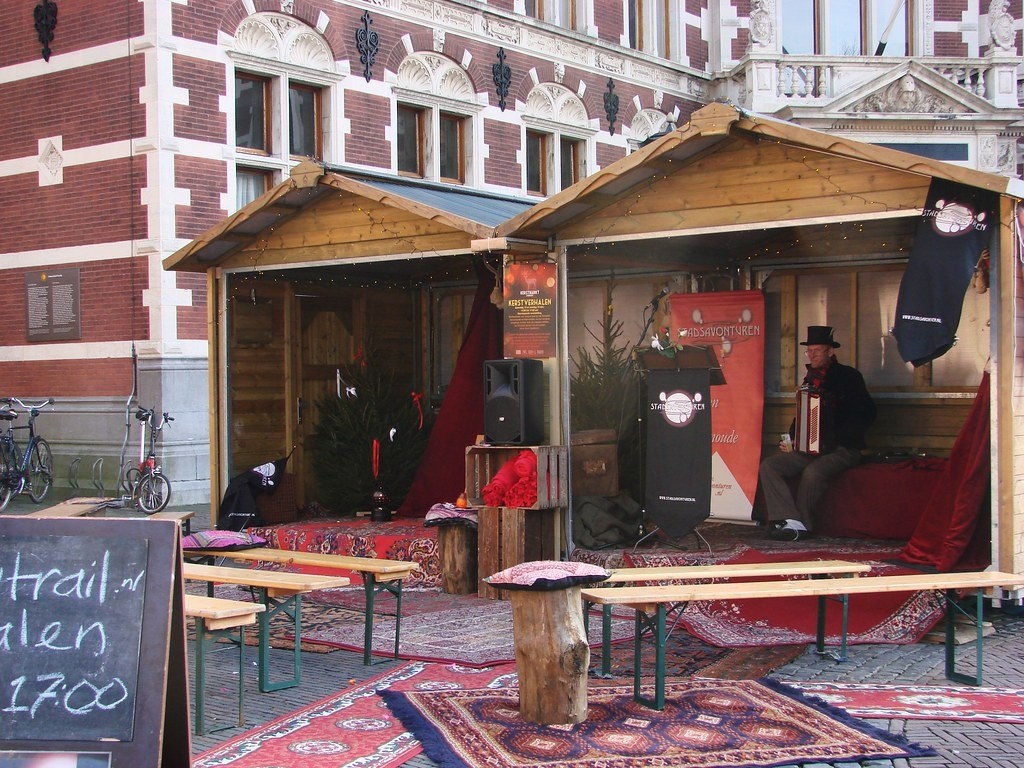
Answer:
[0,537,149,741]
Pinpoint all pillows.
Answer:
[180,528,268,551]
[479,559,613,592]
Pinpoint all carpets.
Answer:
[185,511,1023,768]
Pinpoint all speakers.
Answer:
[483,359,545,446]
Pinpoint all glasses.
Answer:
[804,347,830,357]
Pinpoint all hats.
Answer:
[800,326,841,348]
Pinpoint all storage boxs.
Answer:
[568,429,621,499]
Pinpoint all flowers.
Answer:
[639,325,689,366]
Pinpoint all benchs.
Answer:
[142,512,197,537]
[755,460,951,538]
[180,546,418,668]
[185,596,267,735]
[183,564,349,692]
[26,503,110,516]
[581,559,869,678]
[579,572,1023,711]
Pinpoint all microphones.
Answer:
[645,286,670,310]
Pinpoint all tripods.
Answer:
[590,300,688,552]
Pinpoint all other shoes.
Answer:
[765,521,788,540]
[771,522,809,540]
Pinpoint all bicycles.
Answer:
[0,396,55,514]
[132,406,176,515]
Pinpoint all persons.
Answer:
[758,325,876,540]
